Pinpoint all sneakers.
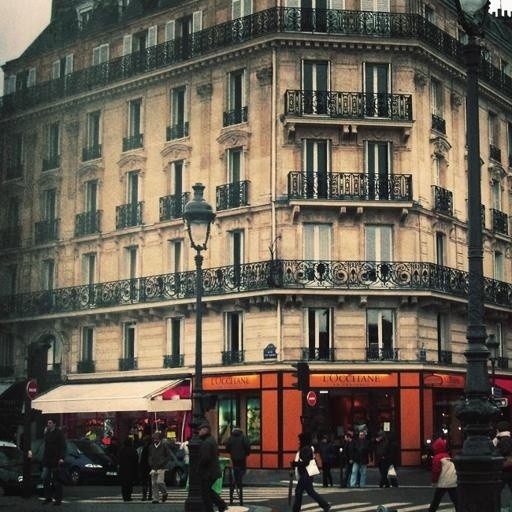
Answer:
[388,466,397,479]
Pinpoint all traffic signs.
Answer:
[491,398,510,410]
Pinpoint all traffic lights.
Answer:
[289,361,309,392]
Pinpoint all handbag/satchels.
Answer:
[305,460,320,476]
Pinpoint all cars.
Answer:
[63,439,120,484]
[0,440,53,495]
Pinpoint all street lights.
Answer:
[448,0,507,512]
[179,182,219,512]
[486,334,501,399]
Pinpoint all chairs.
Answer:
[220,462,249,507]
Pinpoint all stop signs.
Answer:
[25,380,37,401]
[306,390,317,407]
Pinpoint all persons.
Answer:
[428,437,458,512]
[39,418,65,506]
[117,432,191,503]
[225,426,249,487]
[340,430,399,489]
[195,425,227,512]
[289,435,331,512]
[319,436,334,488]
[493,422,512,491]
